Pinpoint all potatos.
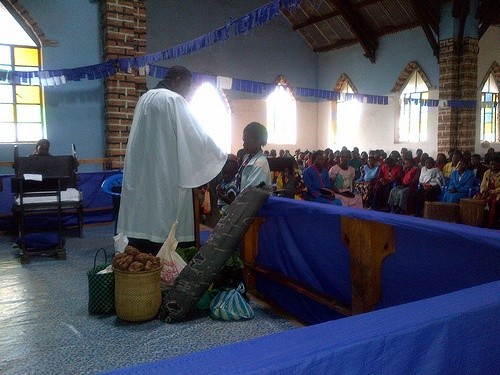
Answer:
[112,246,160,272]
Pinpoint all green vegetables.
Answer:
[177,245,244,290]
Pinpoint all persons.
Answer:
[117,66,240,256]
[199,122,500,228]
[26,139,63,191]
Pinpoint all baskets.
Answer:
[112,263,162,322]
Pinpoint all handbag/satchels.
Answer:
[154,220,188,286]
[210,281,255,320]
[86,248,121,313]
[195,290,219,310]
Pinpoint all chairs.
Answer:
[100,174,123,238]
[13,143,85,240]
[442,178,482,204]
[417,177,450,202]
[9,176,71,264]
